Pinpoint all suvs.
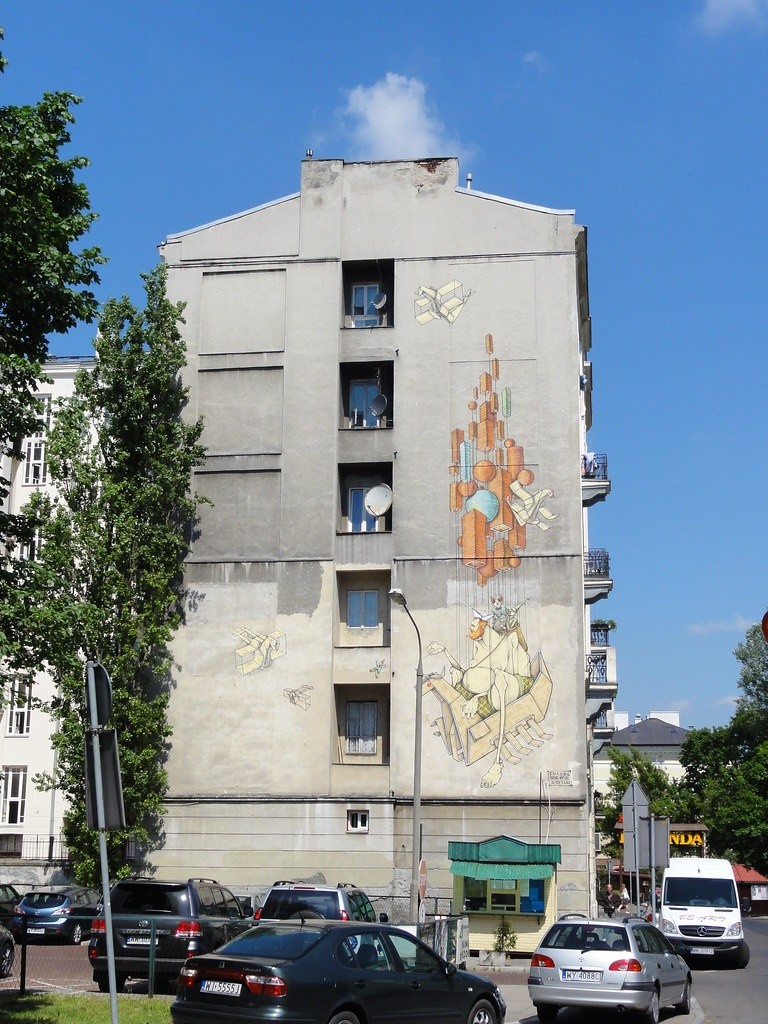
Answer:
[87,876,259,994]
[251,880,389,961]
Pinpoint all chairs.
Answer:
[612,940,623,948]
[580,933,599,949]
[356,944,378,969]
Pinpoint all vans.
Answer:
[653,859,751,971]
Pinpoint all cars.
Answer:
[232,890,269,925]
[170,919,508,1024]
[528,912,693,1024]
[0,923,17,979]
[635,885,662,909]
[0,883,25,931]
[9,884,101,945]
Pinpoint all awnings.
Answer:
[448,834,561,880]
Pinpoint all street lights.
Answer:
[387,587,423,924]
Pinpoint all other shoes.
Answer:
[625,911,629,914]
[617,909,620,913]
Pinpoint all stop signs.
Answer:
[418,859,428,901]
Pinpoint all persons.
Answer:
[620,884,630,909]
[597,885,621,918]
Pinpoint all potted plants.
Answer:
[478,917,519,967]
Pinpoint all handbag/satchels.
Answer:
[623,898,628,904]
